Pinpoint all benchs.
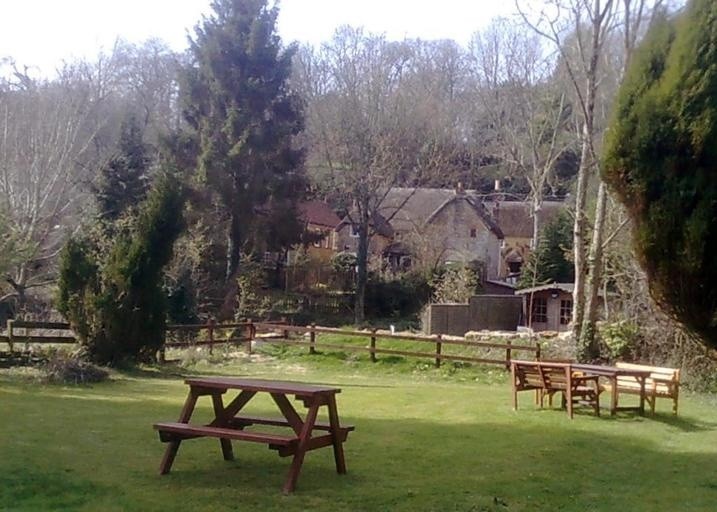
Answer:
[153,416,354,494]
[509,359,681,420]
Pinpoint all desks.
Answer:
[158,371,345,491]
